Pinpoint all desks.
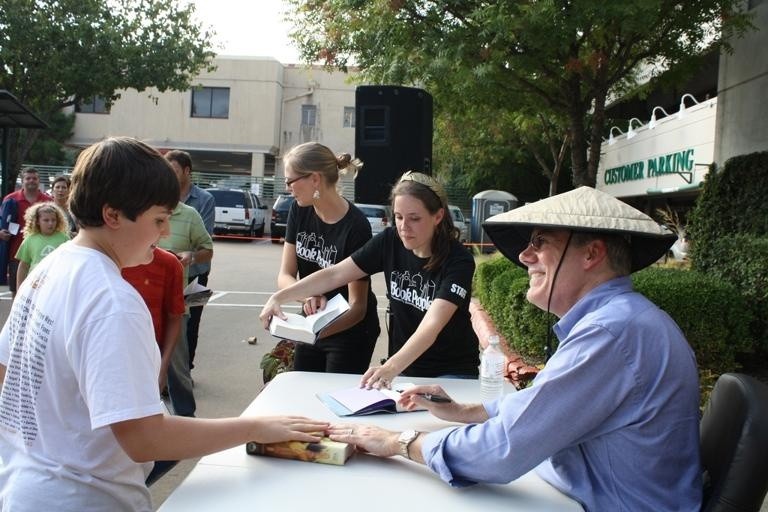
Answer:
[154,371,585,512]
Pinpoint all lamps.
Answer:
[600,94,717,147]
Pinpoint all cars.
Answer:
[660,222,690,261]
[0,176,22,201]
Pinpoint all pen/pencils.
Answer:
[395,388,451,404]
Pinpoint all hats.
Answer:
[483,186,678,272]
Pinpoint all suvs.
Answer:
[447,205,471,245]
[270,193,296,243]
[352,203,393,241]
[202,187,269,242]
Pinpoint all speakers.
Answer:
[354,85,433,206]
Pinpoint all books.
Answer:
[310,381,430,418]
[264,292,353,349]
[244,426,358,465]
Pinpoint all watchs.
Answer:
[397,427,422,462]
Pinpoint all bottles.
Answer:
[480,335,506,410]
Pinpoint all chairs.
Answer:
[698,373,768,512]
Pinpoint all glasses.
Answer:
[285,173,312,187]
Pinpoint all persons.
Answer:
[0,165,54,304]
[0,132,333,512]
[120,242,187,404]
[50,175,80,239]
[322,181,707,511]
[273,139,382,377]
[156,197,215,419]
[163,147,217,388]
[13,200,73,297]
[257,169,484,390]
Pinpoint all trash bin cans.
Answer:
[471,189,519,255]
